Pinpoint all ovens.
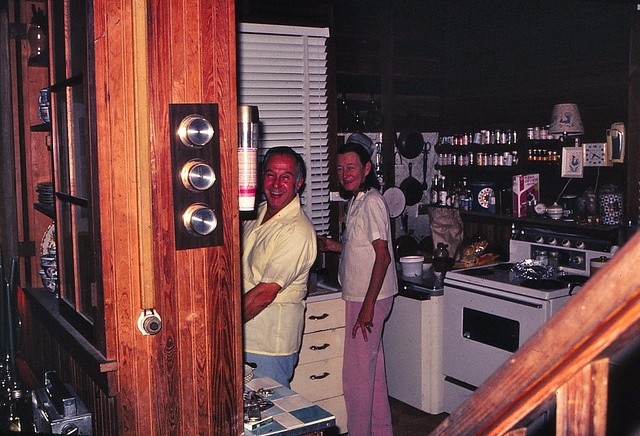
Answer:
[436,286,581,424]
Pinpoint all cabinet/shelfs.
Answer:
[286,291,348,434]
[458,53,639,262]
[327,51,456,266]
[4,1,123,374]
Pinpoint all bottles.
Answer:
[430,173,439,205]
[462,176,467,197]
[433,240,449,276]
[440,175,448,206]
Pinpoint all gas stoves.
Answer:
[442,228,617,301]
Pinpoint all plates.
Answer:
[36,180,56,207]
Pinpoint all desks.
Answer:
[235,364,336,431]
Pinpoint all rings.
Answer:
[366,325,370,328]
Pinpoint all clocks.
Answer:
[581,142,606,168]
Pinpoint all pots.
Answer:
[398,162,423,206]
[395,213,420,261]
[397,131,424,160]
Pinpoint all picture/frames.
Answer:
[560,145,584,180]
[605,123,626,164]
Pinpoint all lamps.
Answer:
[547,103,585,148]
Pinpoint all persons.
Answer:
[238,145,318,397]
[335,144,399,436]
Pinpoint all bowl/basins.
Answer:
[400,255,424,280]
[37,243,59,292]
